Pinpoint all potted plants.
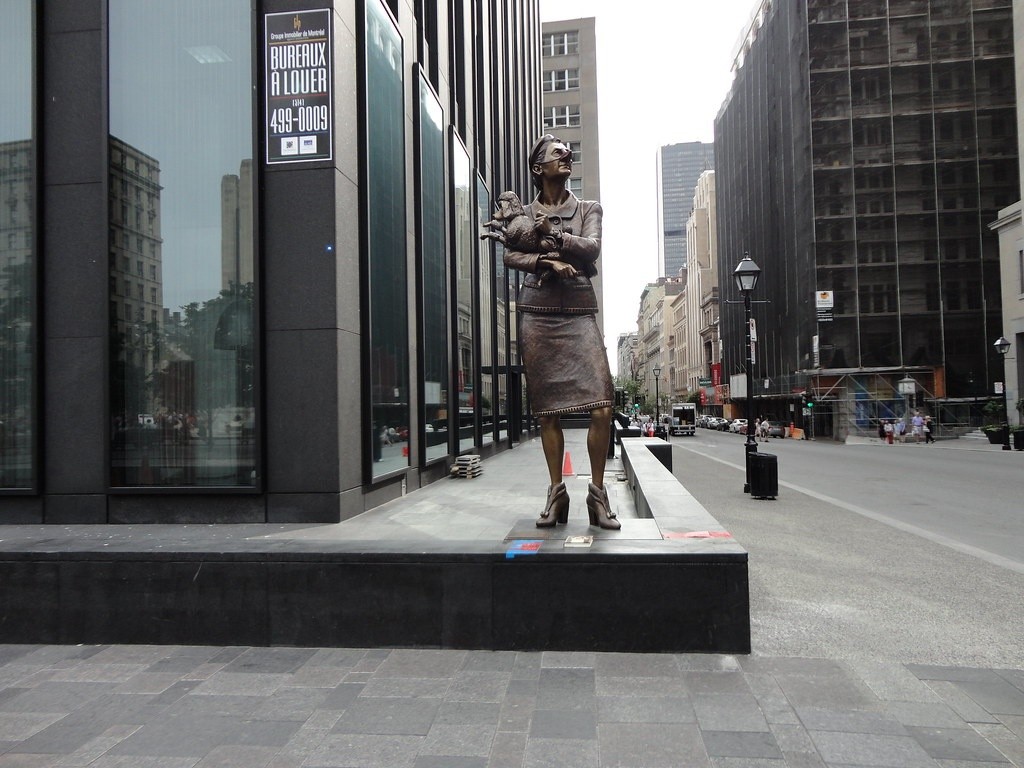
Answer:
[1010,398,1024,449]
[980,401,1009,444]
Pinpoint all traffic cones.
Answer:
[402,446,409,457]
[563,451,575,476]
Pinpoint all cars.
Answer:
[707,417,722,428]
[399,430,409,441]
[700,419,707,428]
[739,423,748,434]
[386,428,400,443]
[716,418,733,431]
[225,414,246,432]
[644,421,667,438]
[425,424,435,432]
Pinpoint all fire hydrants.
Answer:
[791,422,794,436]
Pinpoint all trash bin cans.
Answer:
[747,450,778,497]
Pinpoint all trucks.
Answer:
[458,386,489,415]
[668,403,696,435]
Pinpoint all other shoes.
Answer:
[932,440,936,443]
[916,442,920,444]
[924,442,928,444]
[765,440,769,442]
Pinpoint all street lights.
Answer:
[732,252,760,492]
[653,363,662,437]
[994,337,1011,449]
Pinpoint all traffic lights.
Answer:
[802,393,813,409]
[635,399,638,408]
[631,409,634,414]
[623,390,629,404]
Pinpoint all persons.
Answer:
[630,417,643,434]
[761,417,770,442]
[923,416,936,444]
[504,134,622,529]
[383,425,393,447]
[912,412,923,444]
[647,418,655,437]
[878,418,906,445]
[754,418,762,442]
[167,411,197,438]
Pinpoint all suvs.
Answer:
[729,419,748,433]
[659,414,669,422]
[696,414,712,426]
[757,421,786,438]
